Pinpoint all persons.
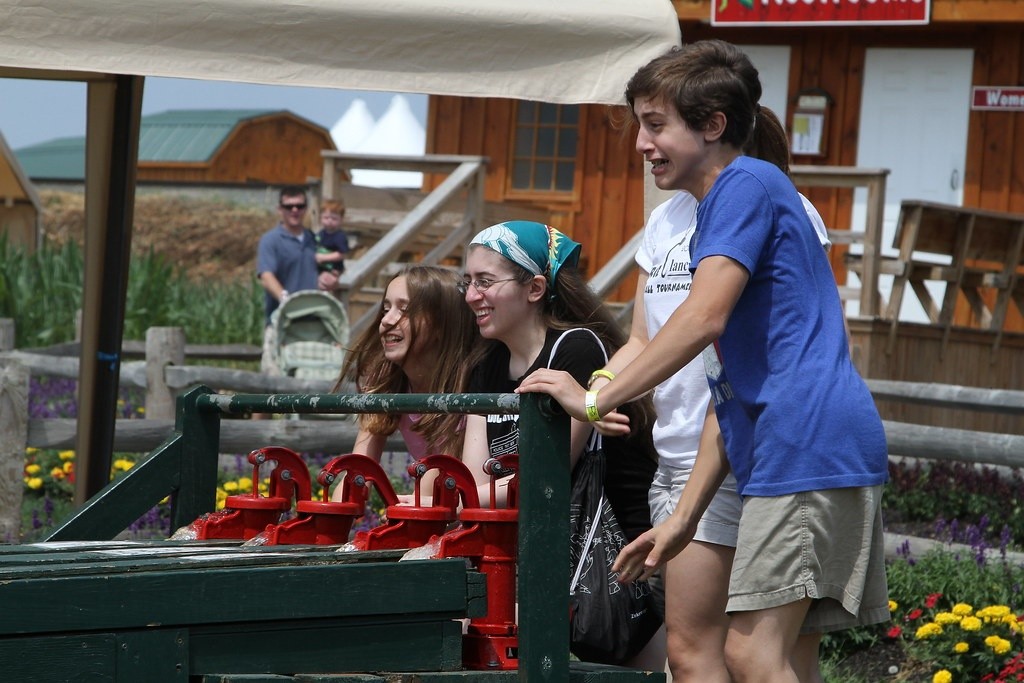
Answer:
[393,36,893,683]
[253,183,351,323]
[329,265,479,506]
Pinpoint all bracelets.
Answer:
[588,369,615,391]
[585,388,605,425]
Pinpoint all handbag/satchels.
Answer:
[547,327,664,665]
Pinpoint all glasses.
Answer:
[281,200,307,210]
[457,277,518,294]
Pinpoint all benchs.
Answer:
[843,198,1024,332]
